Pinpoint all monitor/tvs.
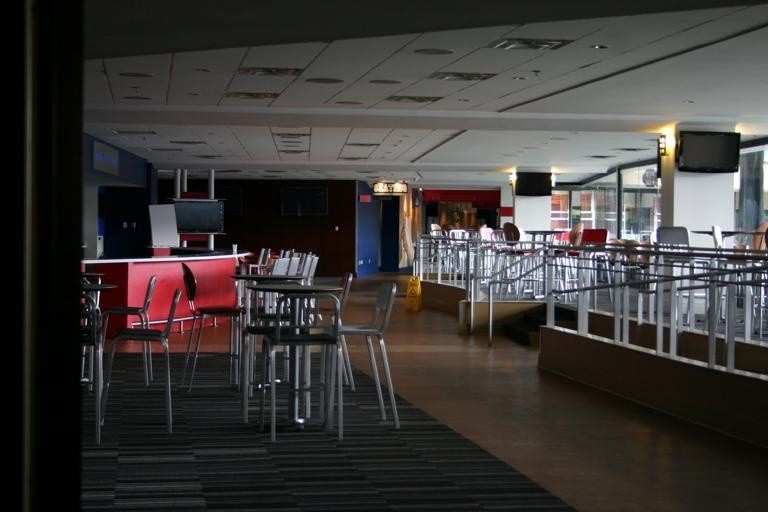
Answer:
[171,198,224,233]
[678,131,741,173]
[515,172,552,196]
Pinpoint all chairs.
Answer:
[76,248,400,446]
[413,223,768,345]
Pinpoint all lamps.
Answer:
[373,180,407,196]
[658,134,669,156]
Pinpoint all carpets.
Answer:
[78,351,577,510]
